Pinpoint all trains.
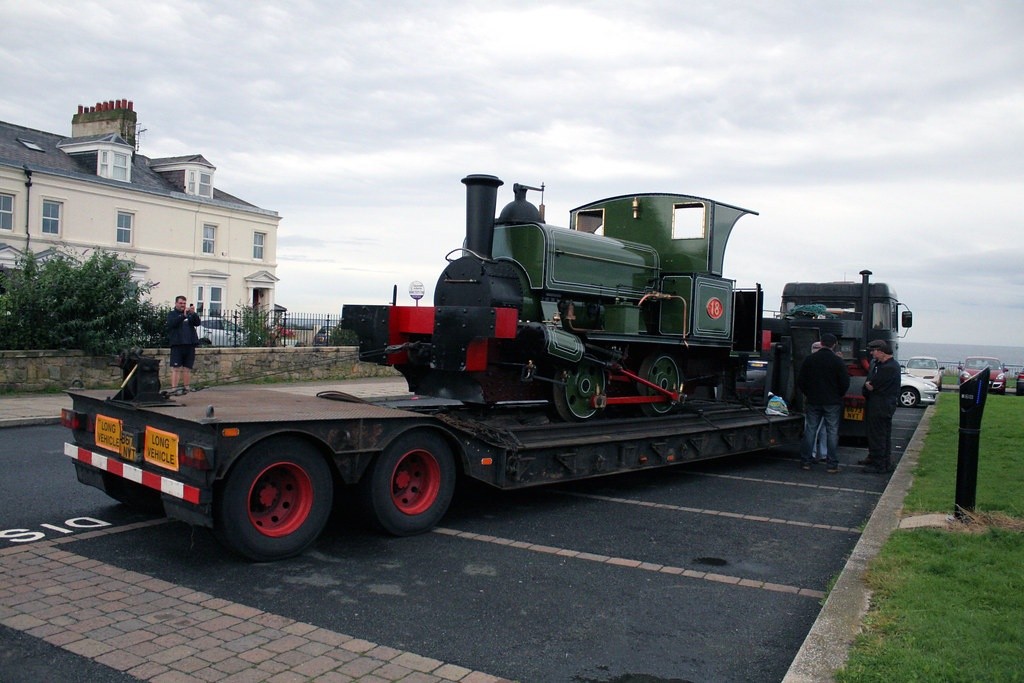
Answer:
[341,174,763,425]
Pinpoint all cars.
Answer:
[957,357,1009,394]
[900,356,946,392]
[188,316,250,347]
[898,368,939,407]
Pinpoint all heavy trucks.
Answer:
[60,271,914,562]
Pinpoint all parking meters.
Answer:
[955,365,990,523]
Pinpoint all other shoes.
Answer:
[858,456,872,465]
[827,464,839,473]
[863,458,886,473]
[885,460,897,472]
[801,462,811,470]
[818,459,827,464]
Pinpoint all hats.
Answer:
[878,344,893,354]
[867,340,886,350]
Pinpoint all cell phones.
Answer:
[190,304,193,307]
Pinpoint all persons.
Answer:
[797,333,850,473]
[805,342,832,463]
[858,340,887,465]
[863,347,901,473]
[167,296,202,390]
